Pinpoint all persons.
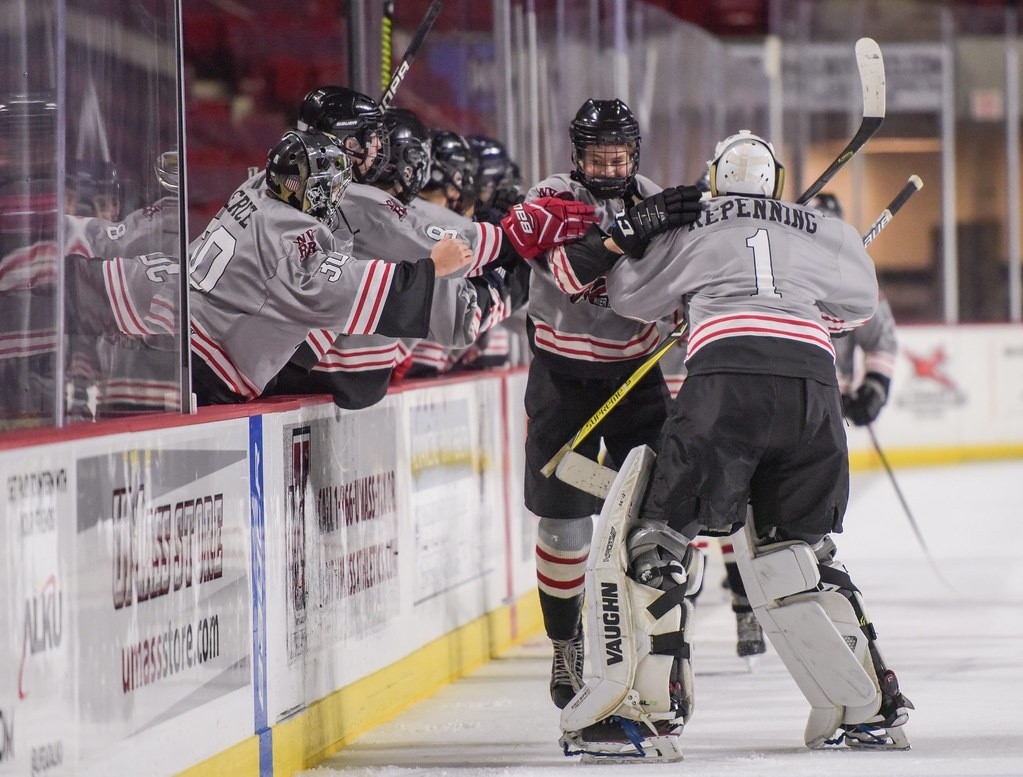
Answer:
[580,127,914,741]
[0,149,179,419]
[519,92,708,732]
[189,84,601,401]
[720,191,893,656]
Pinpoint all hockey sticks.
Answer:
[377,0,442,119]
[867,423,931,560]
[555,174,924,500]
[540,36,888,481]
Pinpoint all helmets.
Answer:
[808,194,844,219]
[264,128,352,219]
[709,128,778,197]
[465,136,521,205]
[566,95,643,194]
[374,109,429,197]
[294,84,388,180]
[0,89,179,221]
[429,131,476,208]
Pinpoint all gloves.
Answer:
[500,196,600,257]
[613,183,704,260]
[846,371,891,425]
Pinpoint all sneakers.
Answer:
[544,629,588,715]
[733,605,767,670]
[832,667,908,749]
[568,680,681,766]
